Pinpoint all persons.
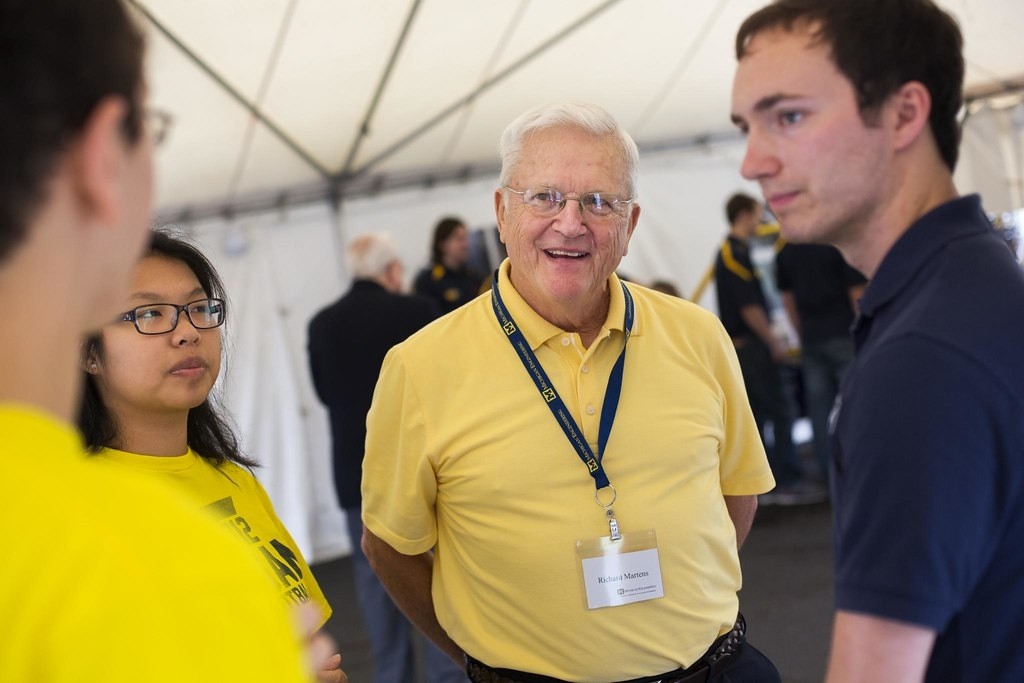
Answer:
[711,194,798,490]
[409,218,479,307]
[73,221,347,683]
[1,0,312,683]
[361,99,783,682]
[729,0,1024,683]
[309,236,449,681]
[774,231,863,478]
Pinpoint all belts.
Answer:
[465,615,744,683]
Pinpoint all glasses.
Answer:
[503,184,633,222]
[118,298,227,336]
[135,107,170,143]
